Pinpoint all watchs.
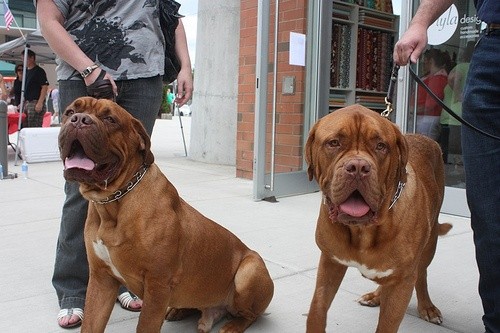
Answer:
[79,63,99,78]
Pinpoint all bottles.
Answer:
[21,161,29,181]
[0,163,4,180]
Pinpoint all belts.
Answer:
[26,100,38,103]
[475,21,500,35]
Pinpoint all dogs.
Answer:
[305,103,453,333]
[58,96,275,333]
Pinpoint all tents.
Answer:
[0,30,188,166]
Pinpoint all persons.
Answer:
[393,0,500,333]
[34,0,193,328]
[0,48,60,129]
[166,88,175,117]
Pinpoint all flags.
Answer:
[2,0,14,31]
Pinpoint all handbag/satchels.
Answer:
[159,0,186,85]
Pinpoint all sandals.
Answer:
[117,291,142,312]
[57,308,84,328]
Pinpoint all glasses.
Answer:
[17,69,23,72]
